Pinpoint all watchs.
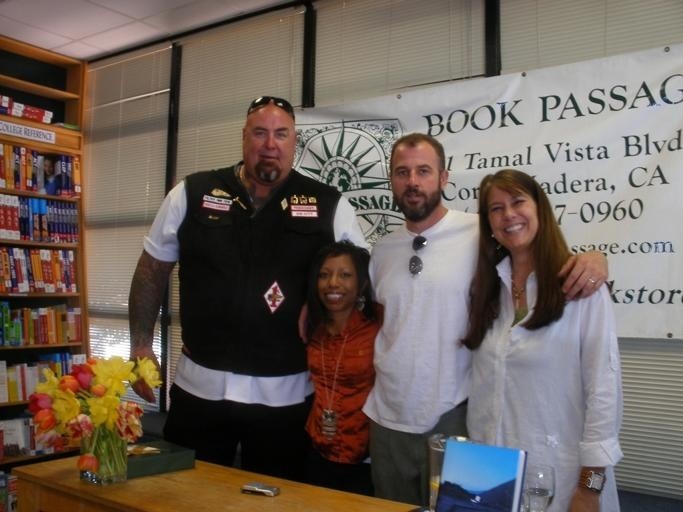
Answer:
[579,470,607,493]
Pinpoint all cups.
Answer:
[523,463,555,512]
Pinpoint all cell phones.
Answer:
[240,482,280,496]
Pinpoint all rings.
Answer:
[589,279,596,284]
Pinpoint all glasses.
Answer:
[409,236,427,277]
[246,95,296,121]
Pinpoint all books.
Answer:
[0,95,88,512]
[434,438,527,511]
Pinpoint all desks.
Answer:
[11,444,425,512]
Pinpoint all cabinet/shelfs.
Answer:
[0,35,91,467]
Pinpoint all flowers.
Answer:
[28,352,165,479]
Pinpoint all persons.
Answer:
[460,170,622,511]
[369,132,608,505]
[297,240,384,495]
[129,96,368,483]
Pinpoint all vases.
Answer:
[79,404,127,485]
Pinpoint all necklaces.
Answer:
[322,334,347,438]
